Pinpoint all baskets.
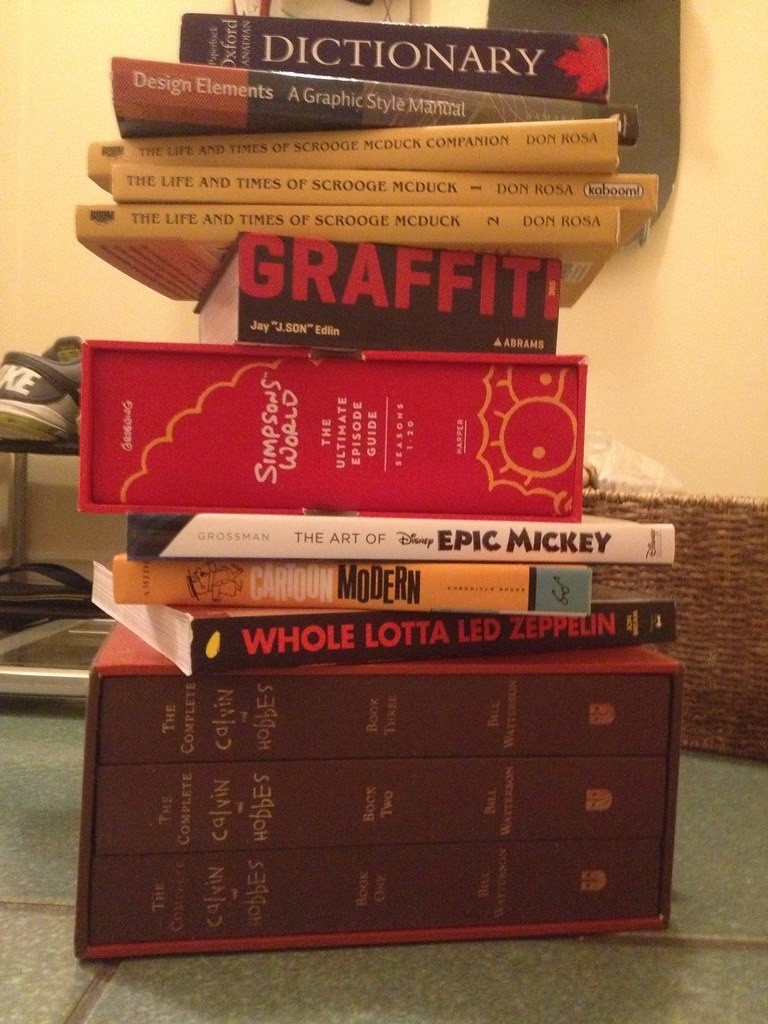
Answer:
[582,462,768,762]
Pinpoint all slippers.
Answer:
[0,562,92,606]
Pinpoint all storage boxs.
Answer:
[70,615,686,965]
[78,340,589,523]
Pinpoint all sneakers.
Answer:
[0,335,83,441]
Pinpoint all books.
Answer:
[73,13,684,959]
